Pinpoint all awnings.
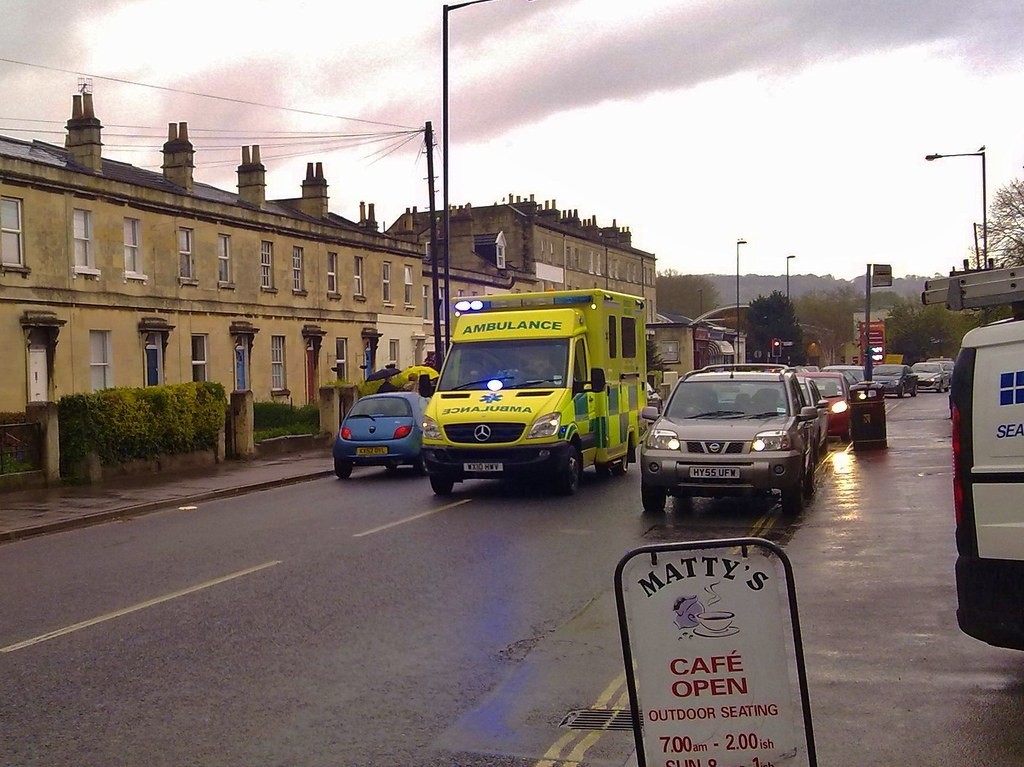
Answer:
[710,340,735,356]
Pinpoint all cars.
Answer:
[796,373,852,442]
[646,382,663,414]
[911,363,950,393]
[872,365,919,398]
[821,365,865,391]
[332,392,431,478]
[936,361,955,385]
[737,377,829,454]
[718,384,740,404]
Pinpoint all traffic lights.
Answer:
[772,338,781,357]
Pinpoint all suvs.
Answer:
[641,363,818,515]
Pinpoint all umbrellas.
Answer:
[366,368,402,381]
[401,363,440,379]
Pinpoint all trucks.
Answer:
[921,266,1024,652]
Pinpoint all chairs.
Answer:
[751,388,779,413]
[689,388,718,412]
[825,381,837,394]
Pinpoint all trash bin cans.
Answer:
[848,382,889,451]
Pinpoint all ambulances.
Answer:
[419,289,648,494]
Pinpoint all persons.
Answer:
[404,372,418,392]
[376,377,398,394]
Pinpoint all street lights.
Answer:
[972,222,982,271]
[787,256,795,299]
[925,152,988,269]
[737,241,747,363]
[698,289,703,315]
